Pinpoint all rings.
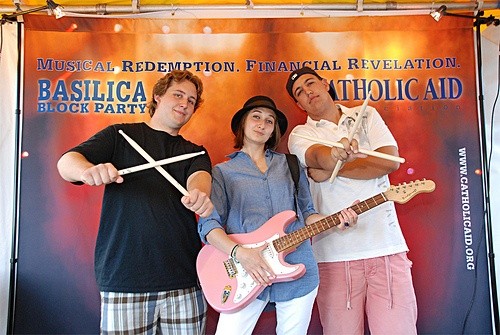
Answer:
[344,221,349,227]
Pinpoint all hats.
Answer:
[231,95,288,137]
[285,67,336,101]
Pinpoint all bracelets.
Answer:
[230,244,239,258]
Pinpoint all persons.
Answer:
[287,67,417,335]
[198,95,361,335]
[57,70,213,335]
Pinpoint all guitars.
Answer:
[196,179,436,314]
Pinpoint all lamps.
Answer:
[431,5,447,22]
[46,0,66,20]
